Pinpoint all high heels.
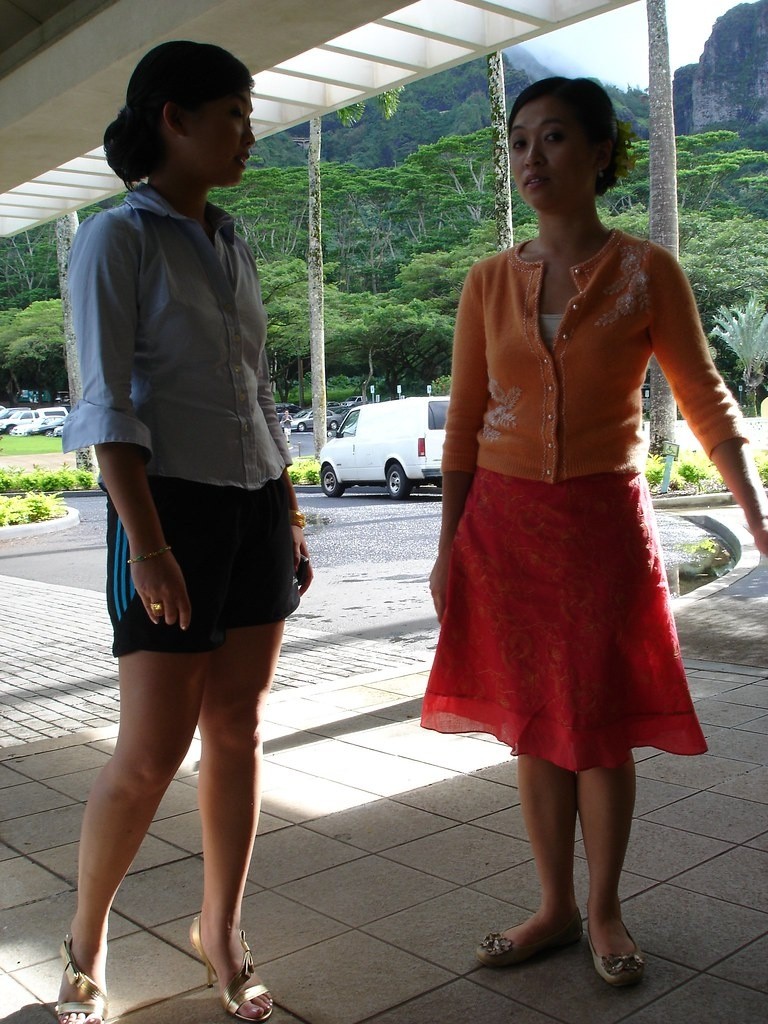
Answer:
[189,916,273,1022]
[55,935,107,1024]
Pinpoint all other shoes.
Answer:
[587,922,643,988]
[476,906,583,968]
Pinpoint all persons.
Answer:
[280,409,293,442]
[55,41,314,1024]
[420,76,768,985]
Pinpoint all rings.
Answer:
[150,603,162,610]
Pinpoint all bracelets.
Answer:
[127,547,171,564]
[289,509,306,529]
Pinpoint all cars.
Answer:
[0,408,32,420]
[36,407,68,419]
[291,409,343,431]
[40,417,66,437]
[18,396,30,403]
[276,404,302,422]
[327,401,340,406]
[53,425,64,437]
[280,410,307,429]
[9,415,65,437]
[0,410,42,435]
[0,406,6,412]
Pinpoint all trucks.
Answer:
[318,397,450,499]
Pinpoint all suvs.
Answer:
[340,395,368,406]
